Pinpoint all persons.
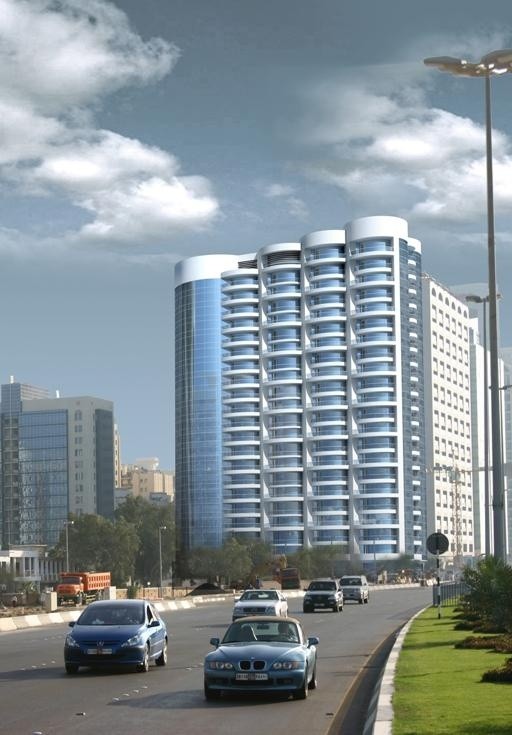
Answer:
[233,624,257,641]
[11,589,105,608]
[270,623,293,641]
[392,574,436,586]
[250,594,257,600]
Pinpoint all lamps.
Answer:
[230,626,296,644]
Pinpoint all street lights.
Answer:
[422,46,512,623]
[157,525,169,585]
[488,384,512,559]
[464,291,503,560]
[62,518,75,572]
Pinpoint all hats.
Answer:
[337,574,370,604]
[64,598,169,674]
[302,578,344,612]
[232,589,288,624]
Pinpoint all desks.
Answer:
[157,525,169,585]
[62,518,75,572]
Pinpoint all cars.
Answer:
[64,598,169,674]
[232,589,288,624]
[302,578,344,612]
[337,574,370,604]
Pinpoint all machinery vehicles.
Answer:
[203,616,320,700]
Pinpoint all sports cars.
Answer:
[203,616,320,700]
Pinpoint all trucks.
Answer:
[279,566,301,590]
[52,568,112,604]
